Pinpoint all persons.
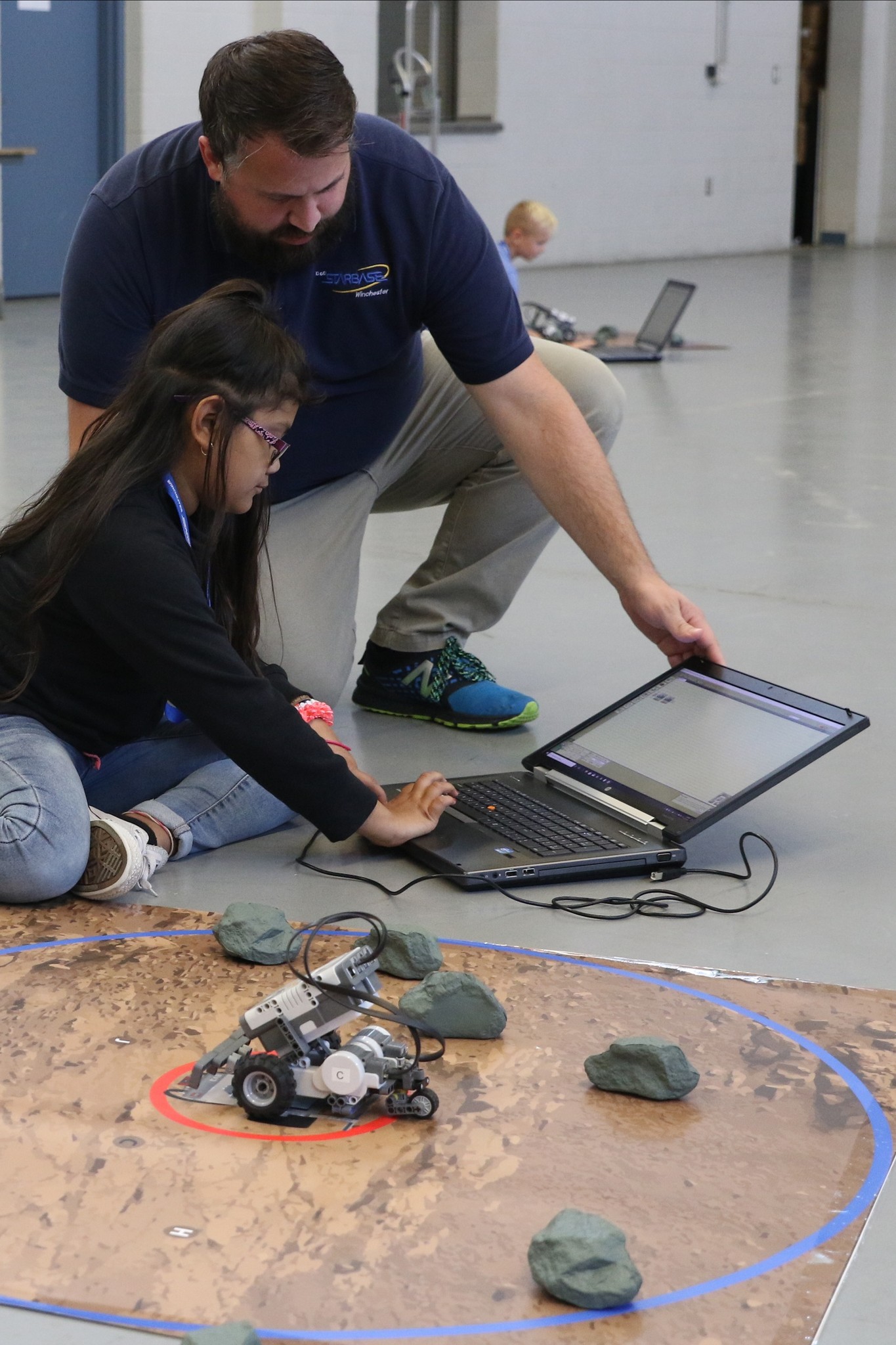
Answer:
[495,200,558,295]
[59,30,722,730]
[0,279,459,902]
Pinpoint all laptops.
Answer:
[378,654,870,890]
[579,279,696,361]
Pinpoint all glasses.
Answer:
[173,394,291,465]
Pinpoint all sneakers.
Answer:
[71,804,168,901]
[351,637,539,730]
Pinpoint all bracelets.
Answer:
[294,699,351,751]
